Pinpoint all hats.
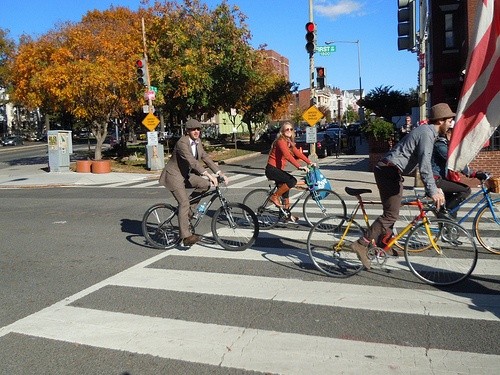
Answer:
[429,102,457,119]
[185,118,201,128]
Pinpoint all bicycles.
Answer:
[307,187,478,287]
[242,162,347,233]
[391,170,500,256]
[142,175,260,252]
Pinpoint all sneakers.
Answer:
[374,247,399,258]
[350,239,371,269]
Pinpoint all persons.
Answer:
[266,122,317,223]
[159,119,229,247]
[349,102,482,269]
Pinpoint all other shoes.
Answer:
[188,208,195,219]
[441,237,463,244]
[440,210,455,220]
[183,234,200,246]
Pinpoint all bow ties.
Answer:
[191,141,199,146]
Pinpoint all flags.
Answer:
[444,0,500,182]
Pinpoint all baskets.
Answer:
[486,177,500,193]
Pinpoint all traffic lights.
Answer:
[316,67,326,78]
[305,22,316,53]
[397,0,415,51]
[135,59,148,86]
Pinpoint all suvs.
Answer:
[75,127,96,143]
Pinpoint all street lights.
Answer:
[325,39,362,108]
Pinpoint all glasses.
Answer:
[189,127,200,131]
[284,128,294,131]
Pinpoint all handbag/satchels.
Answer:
[306,168,331,200]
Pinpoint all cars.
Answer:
[40,132,47,142]
[257,121,361,159]
[2,136,25,147]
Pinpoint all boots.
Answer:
[270,183,291,206]
[279,197,299,222]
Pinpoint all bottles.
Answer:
[192,202,206,219]
